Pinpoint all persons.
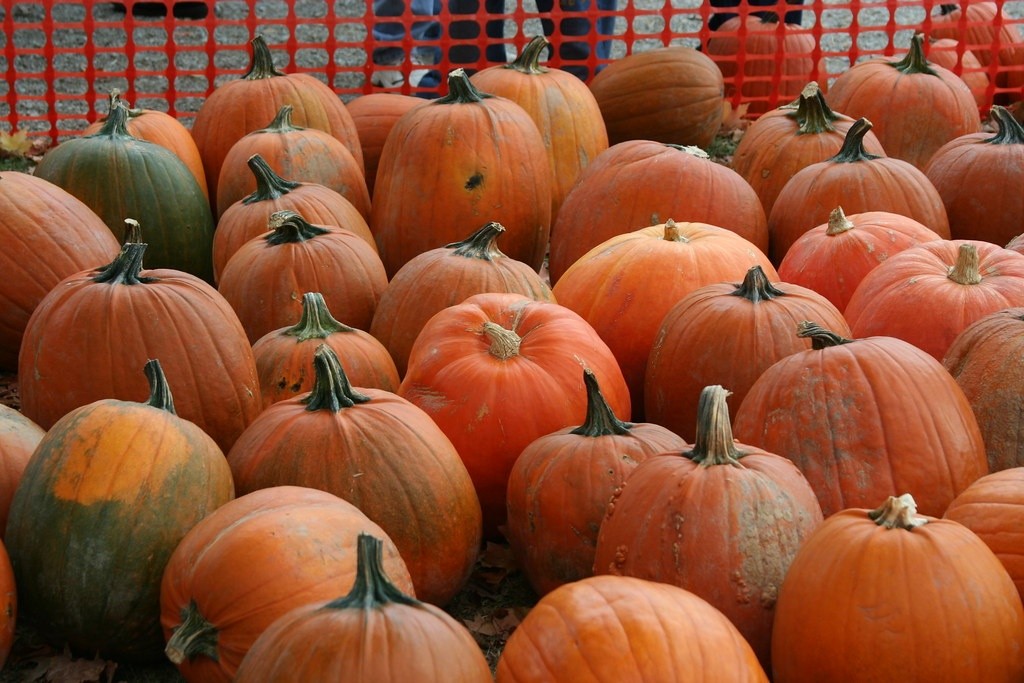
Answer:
[371,0,442,99]
[696,0,804,51]
[436,0,617,82]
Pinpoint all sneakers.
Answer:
[370,55,431,95]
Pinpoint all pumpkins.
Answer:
[0,0,1024,683]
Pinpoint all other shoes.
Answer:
[114,2,208,20]
